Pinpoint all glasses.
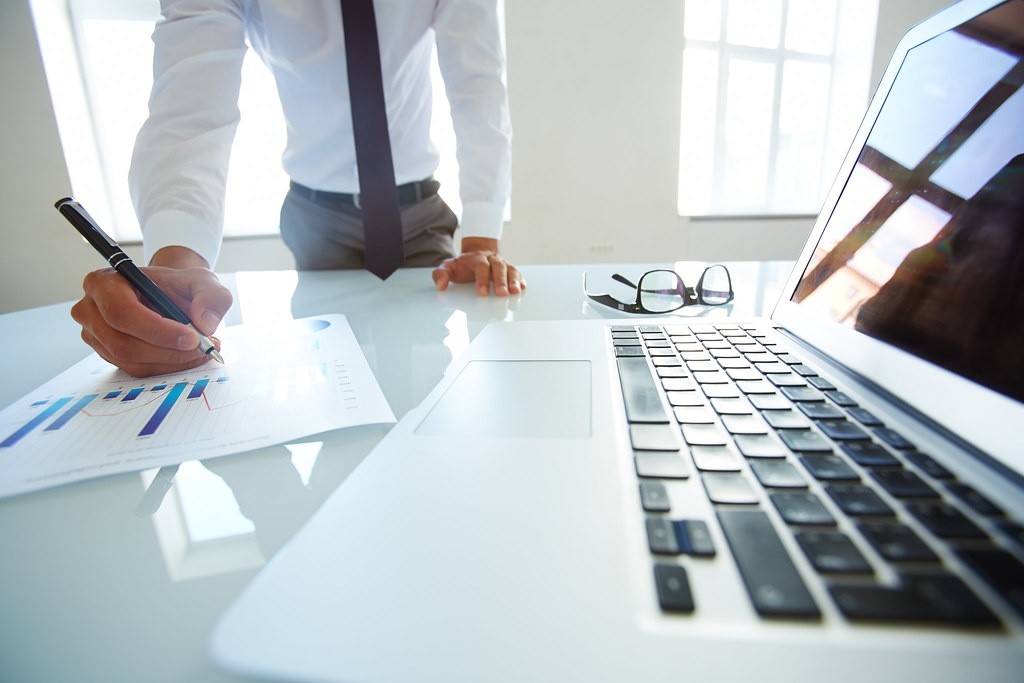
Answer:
[584,265,735,315]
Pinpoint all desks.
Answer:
[0,259,800,682]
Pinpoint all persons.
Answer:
[72,0,525,378]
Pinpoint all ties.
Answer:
[341,0,406,280]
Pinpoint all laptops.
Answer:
[205,0,1024,682]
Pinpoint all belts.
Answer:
[290,176,440,210]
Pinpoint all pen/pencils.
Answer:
[53,196,226,366]
